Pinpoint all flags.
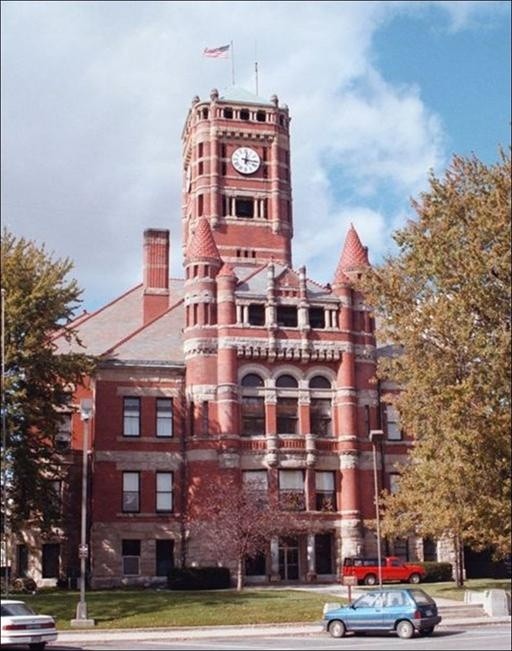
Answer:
[201,44,231,60]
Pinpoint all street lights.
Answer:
[78,398,96,620]
[368,430,388,590]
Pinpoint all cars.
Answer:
[323,590,442,638]
[1,599,58,651]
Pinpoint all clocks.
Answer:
[231,147,262,175]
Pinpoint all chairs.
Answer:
[392,598,399,605]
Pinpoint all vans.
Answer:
[342,555,426,587]
